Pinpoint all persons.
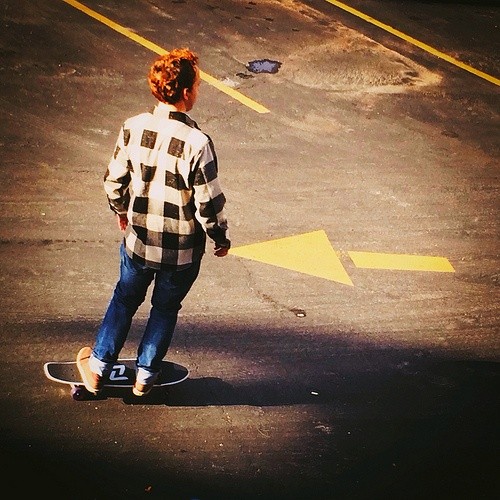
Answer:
[76,48,231,395]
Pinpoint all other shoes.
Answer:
[76,347,106,394]
[132,380,153,396]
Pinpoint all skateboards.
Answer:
[43,358,190,401]
